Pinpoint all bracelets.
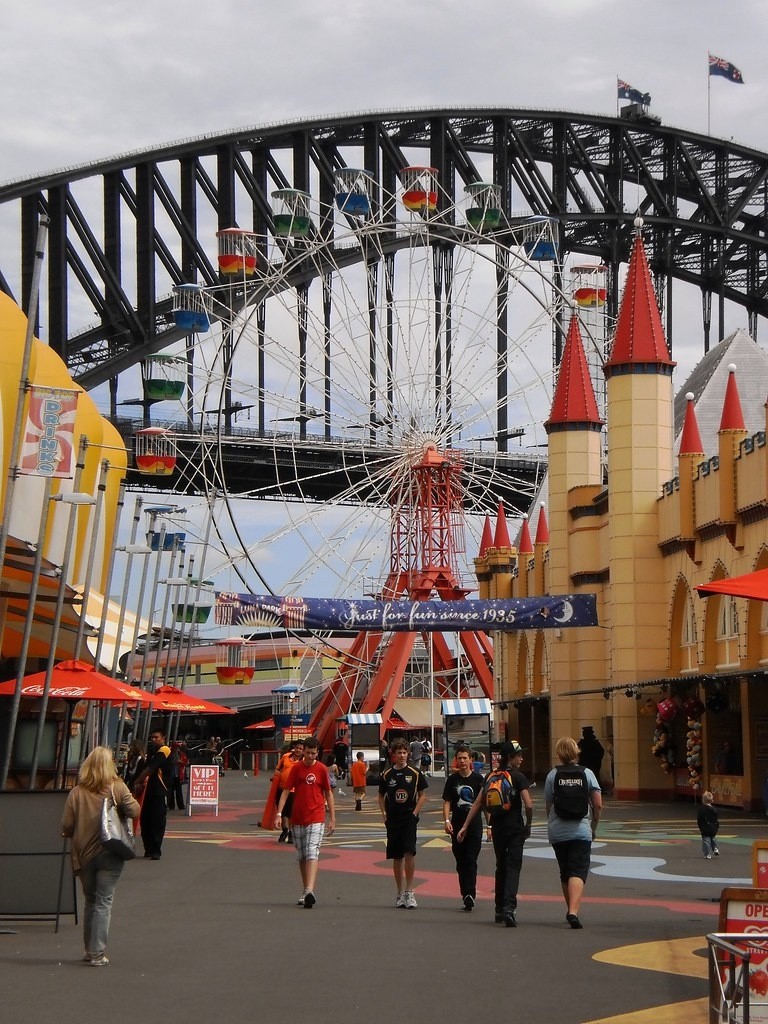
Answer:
[275,812,282,817]
[486,825,492,829]
[445,819,449,823]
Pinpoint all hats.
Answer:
[499,740,526,755]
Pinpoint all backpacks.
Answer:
[554,765,590,819]
[481,769,518,817]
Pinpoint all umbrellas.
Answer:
[93,684,240,747]
[0,530,111,678]
[0,660,168,790]
[243,717,277,730]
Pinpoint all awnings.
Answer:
[490,693,552,707]
[692,568,768,603]
[557,667,768,699]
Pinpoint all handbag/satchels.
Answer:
[101,782,137,861]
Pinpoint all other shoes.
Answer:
[462,895,475,911]
[144,851,161,860]
[496,911,518,926]
[83,952,110,966]
[354,799,363,811]
[299,891,317,909]
[714,847,719,855]
[704,854,712,859]
[566,911,584,928]
[278,829,294,844]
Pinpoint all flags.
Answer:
[618,78,651,107]
[708,55,744,84]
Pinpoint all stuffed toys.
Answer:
[651,711,676,774]
[686,720,703,791]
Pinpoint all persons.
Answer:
[442,738,534,928]
[543,737,603,929]
[408,736,432,776]
[273,737,335,909]
[378,738,430,910]
[57,746,141,966]
[696,791,719,859]
[270,739,348,844]
[123,726,225,860]
[351,752,370,811]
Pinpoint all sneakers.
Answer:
[395,891,418,908]
